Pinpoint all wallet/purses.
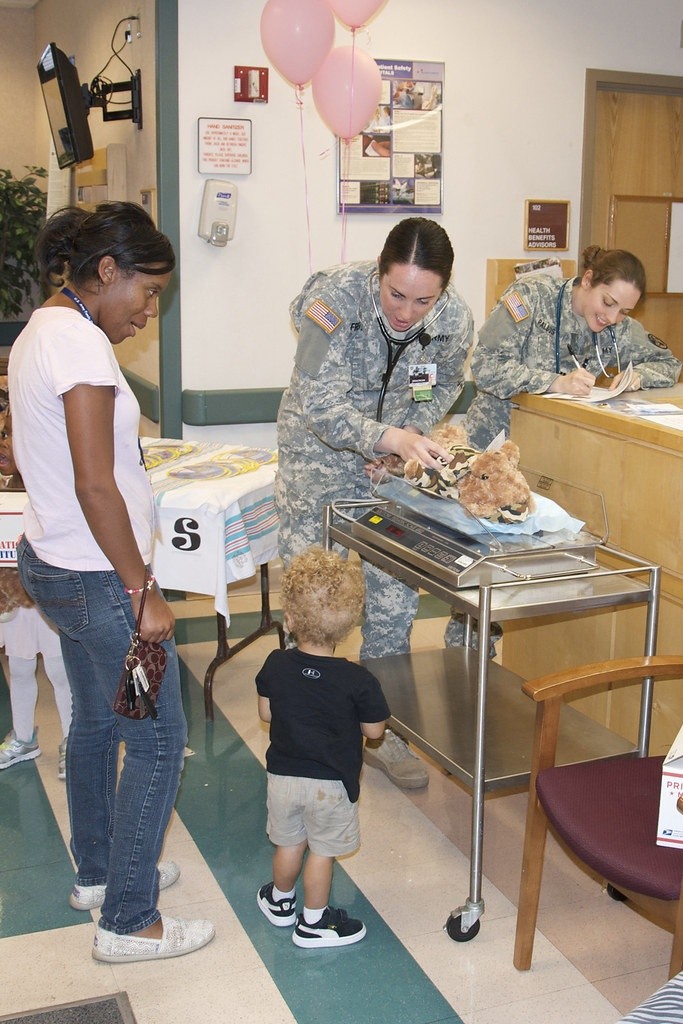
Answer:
[113,565,169,721]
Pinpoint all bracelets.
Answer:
[124,574,156,594]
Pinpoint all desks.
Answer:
[0,438,287,720]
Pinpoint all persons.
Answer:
[257,546,391,949]
[0,411,74,780]
[274,216,473,788]
[366,106,390,130]
[7,201,215,963]
[442,244,683,662]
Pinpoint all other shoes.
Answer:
[92,912,215,962]
[69,859,180,910]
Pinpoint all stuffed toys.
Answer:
[381,421,537,526]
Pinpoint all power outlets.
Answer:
[125,23,133,43]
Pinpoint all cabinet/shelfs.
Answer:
[503,382,683,758]
[322,495,663,935]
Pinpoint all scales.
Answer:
[351,468,609,589]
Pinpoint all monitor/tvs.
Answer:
[38,43,94,171]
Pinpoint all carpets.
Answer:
[0,991,136,1024]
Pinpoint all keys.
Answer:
[126,672,136,710]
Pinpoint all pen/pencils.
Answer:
[566,343,581,368]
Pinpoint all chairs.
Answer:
[514,656,683,980]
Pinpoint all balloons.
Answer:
[260,0,335,89]
[312,45,382,139]
[328,0,384,32]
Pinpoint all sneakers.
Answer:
[256,881,297,927]
[0,726,41,769]
[292,906,366,948]
[58,738,67,779]
[362,729,429,788]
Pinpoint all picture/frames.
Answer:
[525,199,569,251]
